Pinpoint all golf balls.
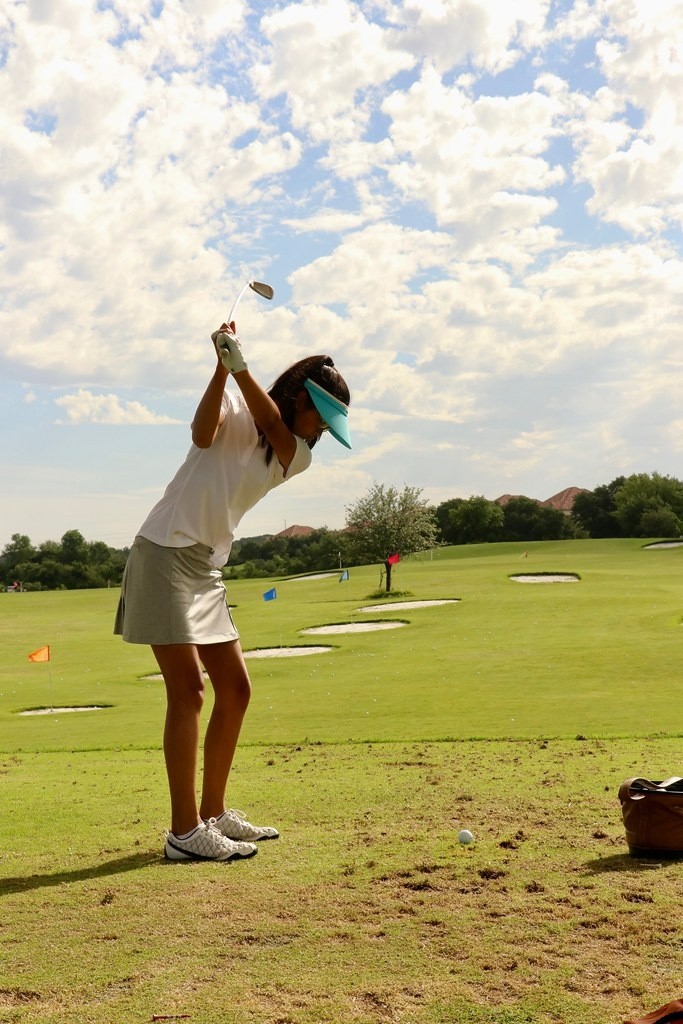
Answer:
[458,830,473,844]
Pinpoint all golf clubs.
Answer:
[218,278,275,359]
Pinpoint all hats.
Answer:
[305,378,352,449]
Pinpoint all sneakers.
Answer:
[198,811,279,840]
[164,820,258,863]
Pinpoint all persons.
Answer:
[114,321,350,859]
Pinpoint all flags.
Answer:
[339,570,349,583]
[389,554,400,564]
[263,588,276,601]
[29,645,50,662]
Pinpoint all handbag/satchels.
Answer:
[618,776,683,859]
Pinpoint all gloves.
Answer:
[216,327,247,375]
